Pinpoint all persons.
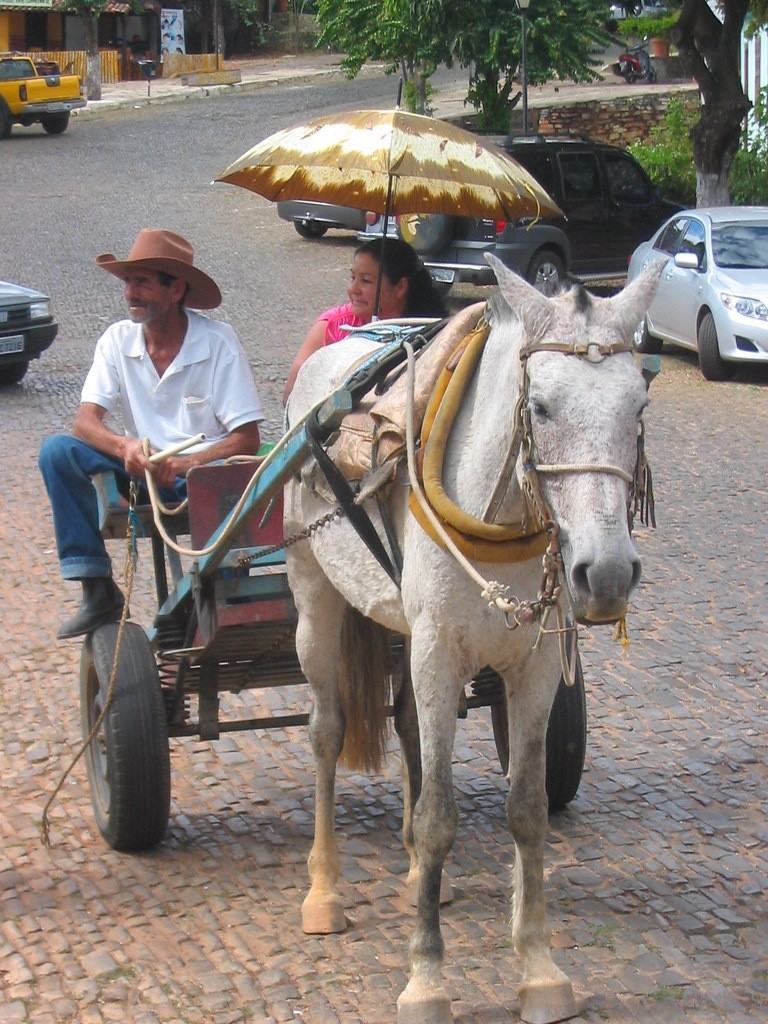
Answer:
[132,34,155,77]
[39,230,264,638]
[283,238,450,408]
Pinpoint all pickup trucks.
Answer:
[0,57,87,139]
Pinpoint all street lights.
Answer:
[515,0,532,133]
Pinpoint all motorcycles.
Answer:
[619,35,657,84]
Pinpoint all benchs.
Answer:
[91,455,191,541]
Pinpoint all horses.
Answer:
[280,249,670,1021]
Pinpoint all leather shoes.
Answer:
[49,579,130,642]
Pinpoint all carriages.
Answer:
[80,252,672,1024]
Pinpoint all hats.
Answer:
[96,226,224,312]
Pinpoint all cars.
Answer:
[625,205,768,381]
[0,280,58,386]
[277,199,368,239]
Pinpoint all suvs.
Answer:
[357,133,687,298]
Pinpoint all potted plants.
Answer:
[648,10,672,58]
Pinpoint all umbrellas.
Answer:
[207,77,570,321]
